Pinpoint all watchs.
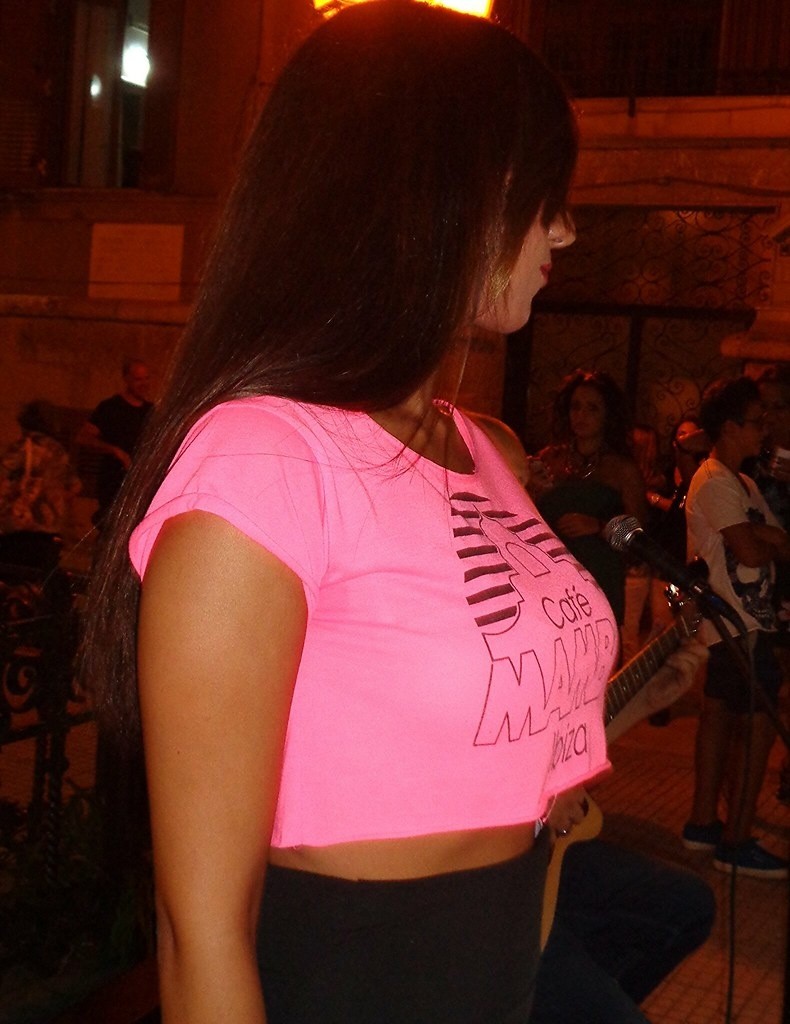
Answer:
[650,494,661,506]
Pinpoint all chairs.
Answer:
[0,532,103,897]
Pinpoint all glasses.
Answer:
[742,411,768,431]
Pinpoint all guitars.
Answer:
[540,590,705,957]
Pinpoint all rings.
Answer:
[561,829,568,835]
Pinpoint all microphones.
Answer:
[600,514,723,621]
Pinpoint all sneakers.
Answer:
[680,819,725,853]
[711,835,790,881]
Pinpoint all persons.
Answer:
[633,418,702,546]
[527,372,643,630]
[69,0,621,1024]
[76,357,155,507]
[682,374,789,879]
